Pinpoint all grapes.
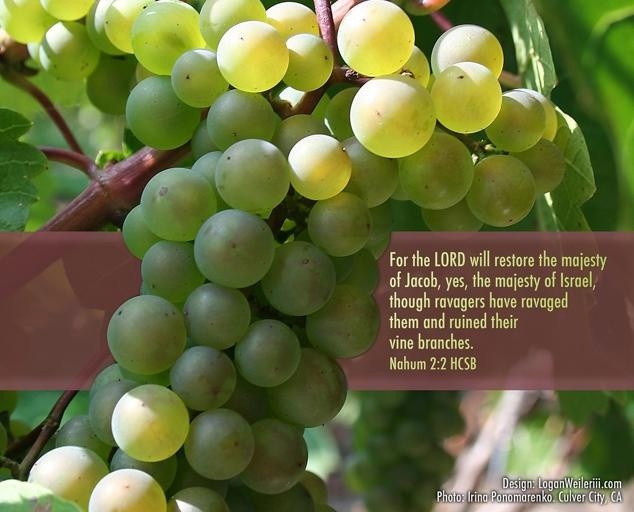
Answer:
[0,0,563,512]
